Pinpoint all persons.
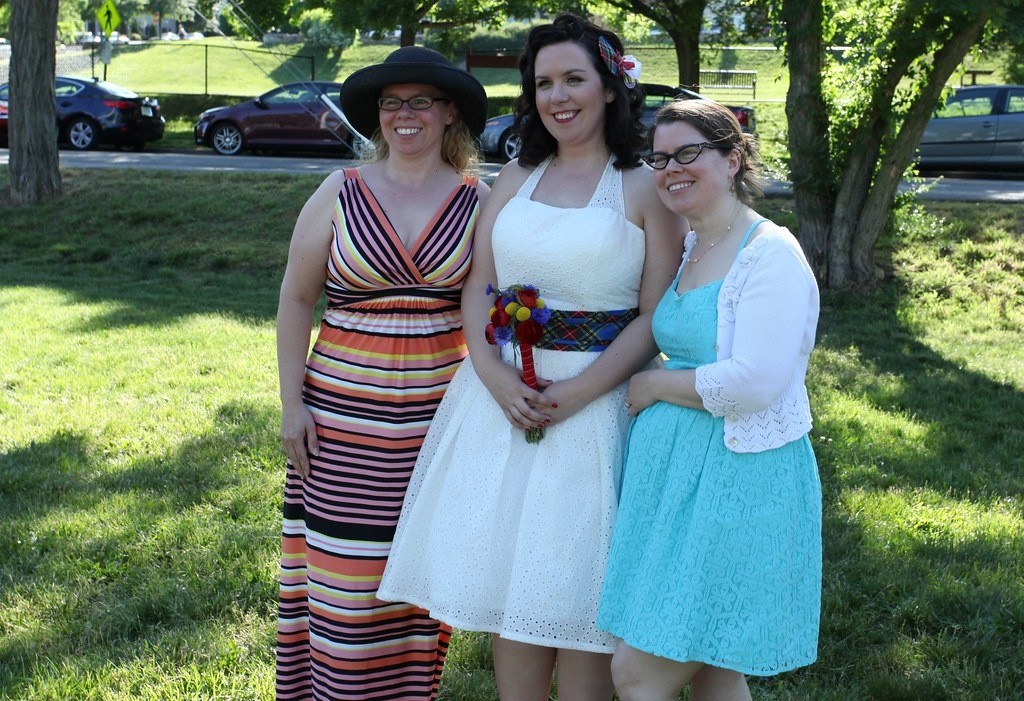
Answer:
[595,99,823,701]
[376,13,690,701]
[275,46,491,701]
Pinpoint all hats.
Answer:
[339,45,487,143]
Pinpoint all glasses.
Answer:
[378,95,446,111]
[642,142,727,169]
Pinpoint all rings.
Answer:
[625,403,630,408]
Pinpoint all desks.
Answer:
[955,67,996,86]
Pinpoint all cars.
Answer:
[478,83,755,164]
[150,31,205,40]
[78,30,128,49]
[912,85,1024,178]
[194,82,376,159]
[0,76,165,153]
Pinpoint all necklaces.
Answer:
[686,207,737,263]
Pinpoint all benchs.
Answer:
[697,69,757,100]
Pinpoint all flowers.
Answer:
[485,283,554,446]
